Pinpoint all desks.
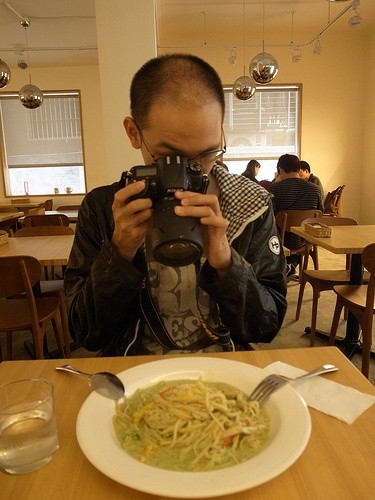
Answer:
[0,234,78,360]
[18,210,78,280]
[0,203,42,216]
[0,212,24,232]
[0,347,375,500]
[288,223,375,361]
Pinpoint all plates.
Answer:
[75,356,312,497]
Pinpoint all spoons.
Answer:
[55,363,125,400]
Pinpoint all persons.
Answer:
[241,160,261,184]
[258,155,325,284]
[62,53,287,356]
[299,161,325,199]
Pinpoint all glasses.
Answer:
[132,119,227,168]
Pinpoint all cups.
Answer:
[54,188,59,194]
[64,186,72,194]
[0,378,60,476]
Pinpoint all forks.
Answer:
[248,363,338,405]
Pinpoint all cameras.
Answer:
[125,154,209,267]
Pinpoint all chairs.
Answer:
[276,210,375,378]
[0,199,81,362]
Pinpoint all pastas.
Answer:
[116,375,270,467]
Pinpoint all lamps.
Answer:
[312,37,320,56]
[228,47,238,67]
[348,0,362,28]
[290,44,302,62]
[15,50,27,69]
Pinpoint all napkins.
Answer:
[264,361,375,425]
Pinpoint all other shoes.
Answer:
[287,270,296,277]
